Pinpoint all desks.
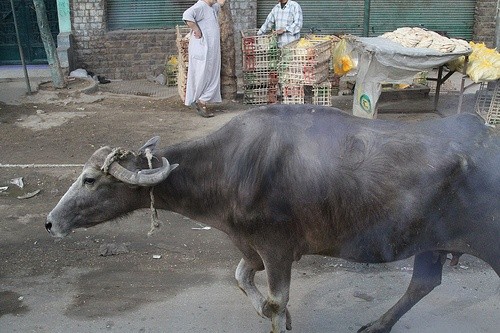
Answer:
[345,36,472,123]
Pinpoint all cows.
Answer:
[44,103,500,333]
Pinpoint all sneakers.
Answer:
[190,102,215,117]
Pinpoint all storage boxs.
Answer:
[240,27,335,111]
[175,23,202,104]
[472,76,500,126]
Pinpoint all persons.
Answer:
[181,0,226,117]
[256,0,304,50]
